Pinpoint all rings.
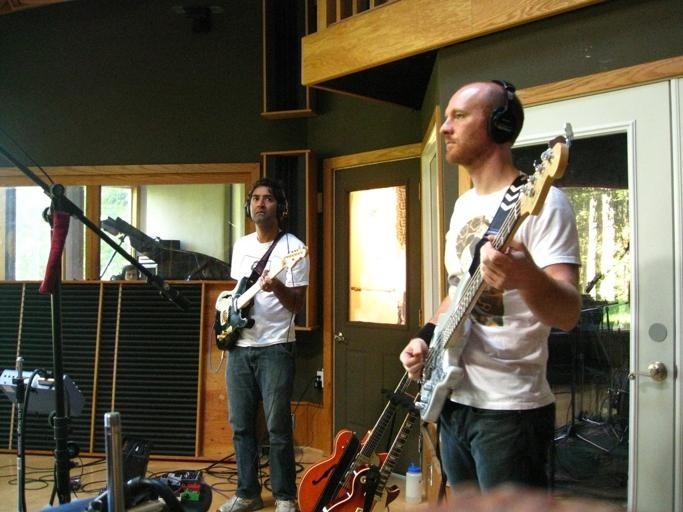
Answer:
[265,286,268,290]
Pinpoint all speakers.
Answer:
[261,0,316,121]
[260,148,317,334]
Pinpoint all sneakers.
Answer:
[274,499,298,512]
[216,494,264,512]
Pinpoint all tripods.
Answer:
[553,327,610,453]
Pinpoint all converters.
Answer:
[314,381,321,388]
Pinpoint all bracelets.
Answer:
[415,323,436,349]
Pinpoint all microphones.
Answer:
[169,290,192,311]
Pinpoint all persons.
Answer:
[216,179,310,512]
[121,265,140,280]
[398,80,581,493]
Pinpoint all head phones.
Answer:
[245,177,289,221]
[488,78,519,144]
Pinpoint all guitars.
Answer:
[216,246,309,350]
[297,372,420,512]
[414,124,573,422]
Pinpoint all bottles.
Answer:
[406,463,422,505]
